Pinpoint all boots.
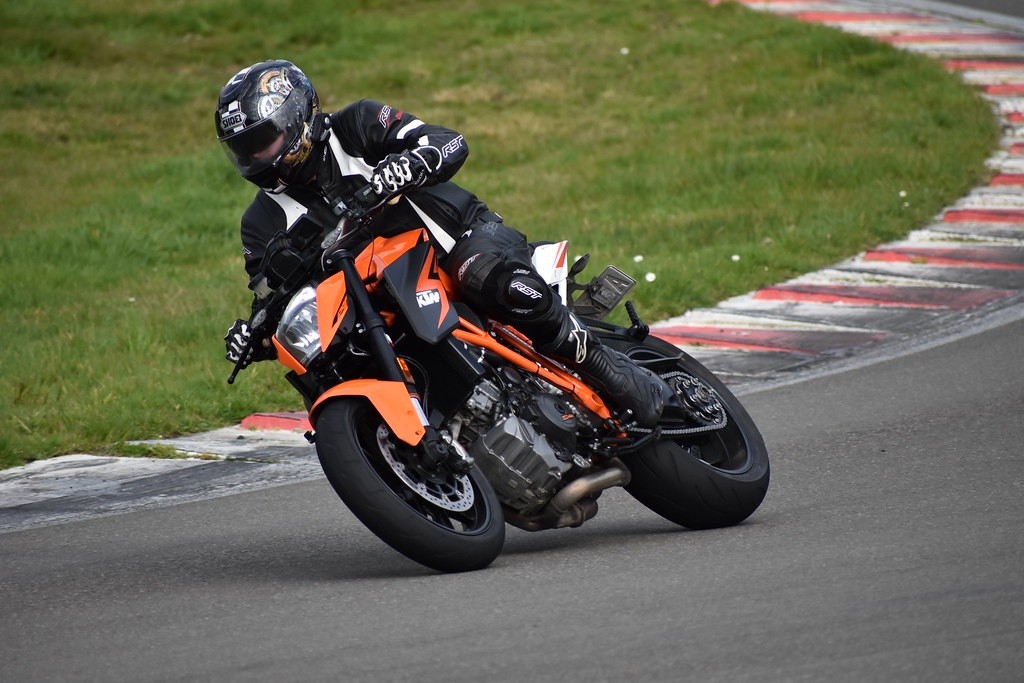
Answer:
[532,305,661,429]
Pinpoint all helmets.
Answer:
[214,59,320,195]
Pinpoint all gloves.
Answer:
[370,149,437,197]
[223,318,271,370]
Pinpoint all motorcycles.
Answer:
[225,182,771,575]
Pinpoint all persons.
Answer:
[213,58,665,429]
